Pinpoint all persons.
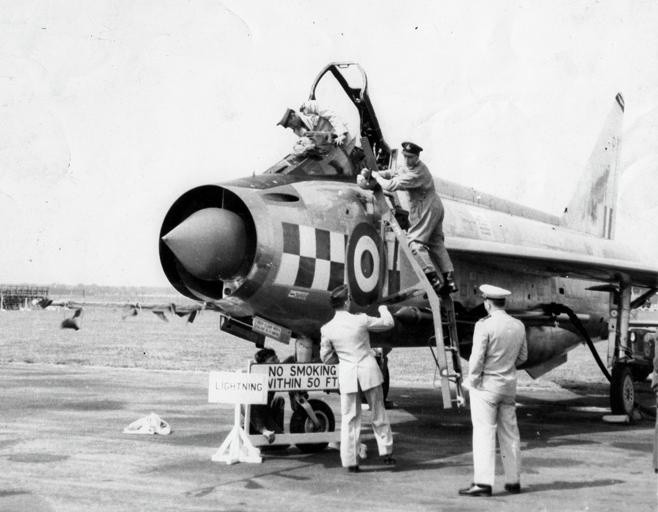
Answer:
[458,283,529,497]
[361,141,459,300]
[318,282,397,473]
[274,99,358,176]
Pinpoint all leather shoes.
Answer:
[348,466,360,473]
[384,457,396,464]
[505,483,520,494]
[459,483,492,497]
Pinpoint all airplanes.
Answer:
[29,60,658,456]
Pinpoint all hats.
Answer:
[331,285,349,299]
[479,284,512,299]
[277,109,291,128]
[255,348,275,363]
[402,142,423,156]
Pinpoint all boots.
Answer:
[423,271,459,299]
